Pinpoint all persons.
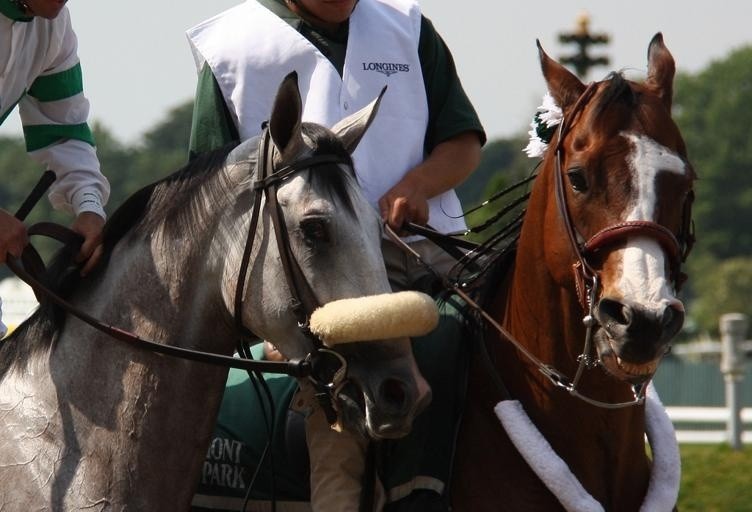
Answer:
[185,0,487,292]
[0,0,110,284]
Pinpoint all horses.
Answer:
[0,70,432,512]
[188,31,701,512]
